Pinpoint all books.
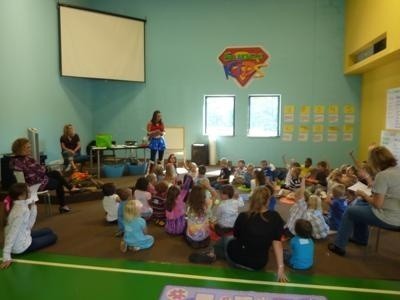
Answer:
[345,183,373,199]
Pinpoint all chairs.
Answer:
[14,169,52,218]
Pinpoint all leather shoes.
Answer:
[327,242,346,257]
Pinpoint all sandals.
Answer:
[188,251,217,266]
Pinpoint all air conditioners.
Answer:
[27,126,40,165]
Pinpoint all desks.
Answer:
[90,144,149,178]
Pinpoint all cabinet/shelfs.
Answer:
[3,153,47,188]
[191,144,209,166]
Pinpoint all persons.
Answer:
[152,164,164,181]
[189,188,290,283]
[325,167,340,188]
[350,149,368,188]
[260,162,269,170]
[183,150,198,181]
[131,178,153,222]
[245,163,254,186]
[9,138,85,213]
[236,160,246,177]
[147,110,166,163]
[0,183,58,268]
[283,219,316,272]
[120,199,154,253]
[251,170,274,192]
[142,174,156,196]
[302,195,330,241]
[218,158,230,177]
[339,166,356,183]
[101,183,124,224]
[263,184,275,213]
[227,161,234,175]
[167,155,177,175]
[274,171,285,189]
[194,165,209,186]
[60,124,81,174]
[185,181,216,250]
[282,178,307,239]
[150,181,168,227]
[113,189,133,237]
[214,169,229,188]
[303,175,322,199]
[286,161,300,186]
[318,161,329,177]
[288,168,305,204]
[166,169,192,236]
[260,160,272,181]
[327,145,399,256]
[340,174,358,207]
[212,185,244,235]
[300,158,314,179]
[323,184,347,231]
